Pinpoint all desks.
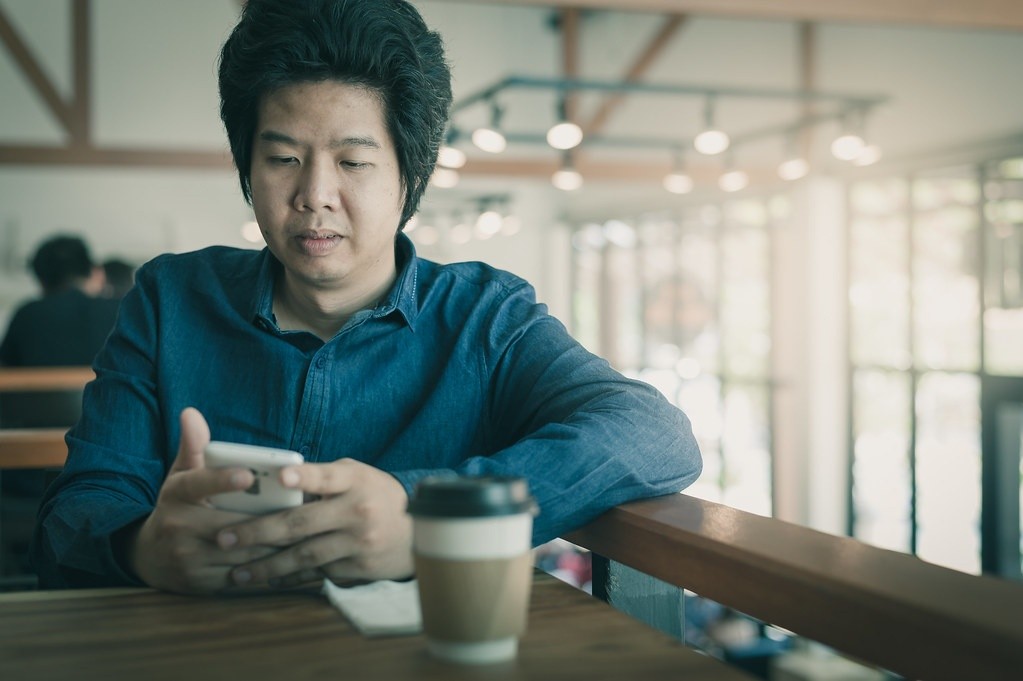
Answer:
[0,564,760,681]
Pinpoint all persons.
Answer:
[0,234,143,499]
[35,0,701,594]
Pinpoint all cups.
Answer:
[406,475,541,662]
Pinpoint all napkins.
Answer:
[321,576,422,639]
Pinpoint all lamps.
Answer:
[693,99,730,155]
[472,106,509,154]
[551,149,584,192]
[438,128,467,169]
[546,101,584,149]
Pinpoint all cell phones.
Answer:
[205,441,304,514]
